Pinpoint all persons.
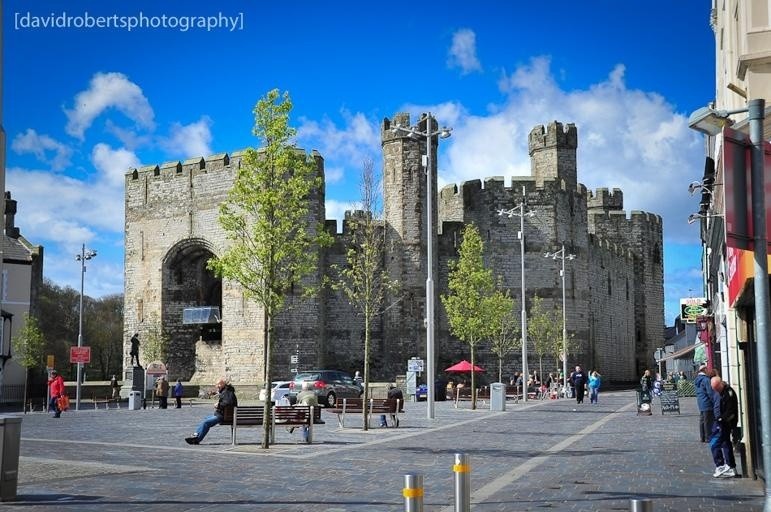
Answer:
[285,382,318,442]
[47,371,65,418]
[572,366,586,403]
[637,367,687,406]
[379,384,403,427]
[587,369,602,405]
[155,377,170,409]
[174,380,184,408]
[444,359,486,389]
[508,366,576,399]
[110,375,118,399]
[185,378,237,444]
[695,365,738,478]
[130,333,142,367]
[352,371,362,392]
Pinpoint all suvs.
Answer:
[289,371,365,406]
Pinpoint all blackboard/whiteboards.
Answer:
[660,390,679,412]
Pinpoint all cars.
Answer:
[260,379,293,404]
[416,379,455,402]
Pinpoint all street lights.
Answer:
[71,243,97,410]
[495,203,540,401]
[544,244,576,399]
[387,112,453,419]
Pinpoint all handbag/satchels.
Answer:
[58,397,69,410]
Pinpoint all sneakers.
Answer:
[53,411,61,418]
[286,427,293,433]
[380,424,387,427]
[185,437,199,445]
[713,465,737,477]
[301,437,308,442]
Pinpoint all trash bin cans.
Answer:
[490,382,506,411]
[129,391,141,411]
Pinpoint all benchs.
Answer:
[452,386,522,408]
[326,398,404,427]
[220,405,324,444]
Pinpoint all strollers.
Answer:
[635,389,651,416]
